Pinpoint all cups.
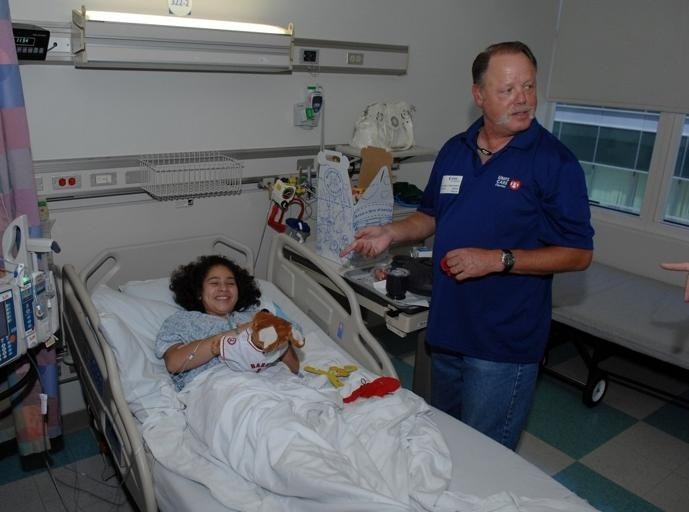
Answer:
[382,266,410,299]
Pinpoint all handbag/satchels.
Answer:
[352,100,417,153]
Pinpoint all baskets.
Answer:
[144,152,244,199]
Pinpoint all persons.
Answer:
[662,262,689,304]
[339,41,595,449]
[156,256,300,386]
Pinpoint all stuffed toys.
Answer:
[210,307,306,373]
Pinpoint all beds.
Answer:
[60,233,596,510]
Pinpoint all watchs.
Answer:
[500,248,515,273]
